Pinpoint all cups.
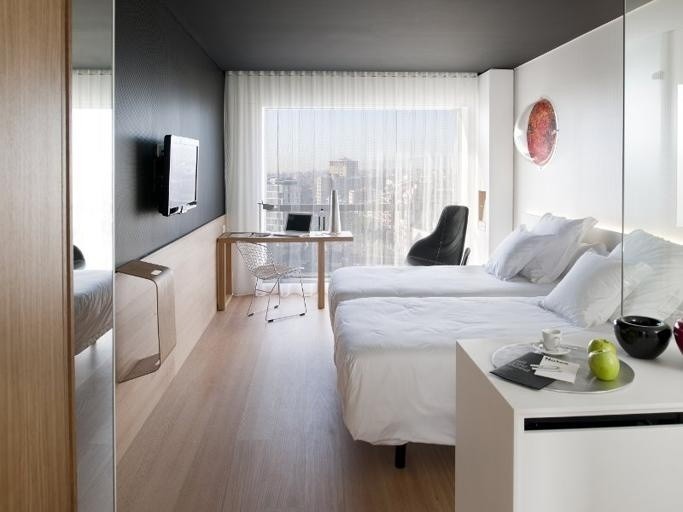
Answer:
[543,330,561,350]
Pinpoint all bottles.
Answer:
[318,209,325,234]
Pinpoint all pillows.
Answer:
[606,228,682,329]
[519,213,599,284]
[485,223,556,283]
[539,246,649,329]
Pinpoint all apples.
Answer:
[588,349,620,381]
[587,338,617,354]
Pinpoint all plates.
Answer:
[540,346,572,355]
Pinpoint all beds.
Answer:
[335,227,683,468]
[329,213,622,331]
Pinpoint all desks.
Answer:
[217,230,354,311]
[453,338,682,512]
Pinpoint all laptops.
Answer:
[274,212,313,238]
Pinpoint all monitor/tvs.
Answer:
[156,134,201,217]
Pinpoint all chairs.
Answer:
[407,205,469,266]
[460,247,472,267]
[237,240,308,323]
